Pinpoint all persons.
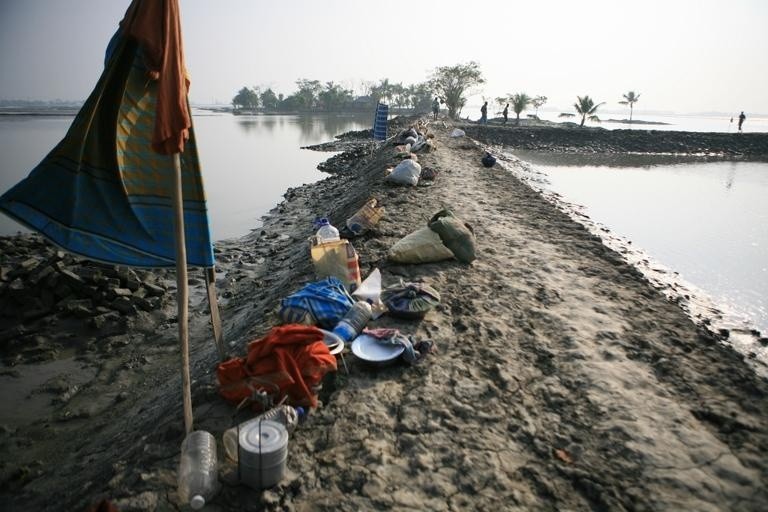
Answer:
[476,101,488,125]
[738,111,746,132]
[501,103,510,126]
[431,97,439,121]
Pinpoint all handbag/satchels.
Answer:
[309,238,361,292]
[280,275,355,323]
[216,322,338,407]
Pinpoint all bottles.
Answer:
[177,431,217,508]
[333,296,374,340]
[315,216,341,246]
[223,406,306,462]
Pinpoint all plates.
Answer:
[351,333,407,362]
